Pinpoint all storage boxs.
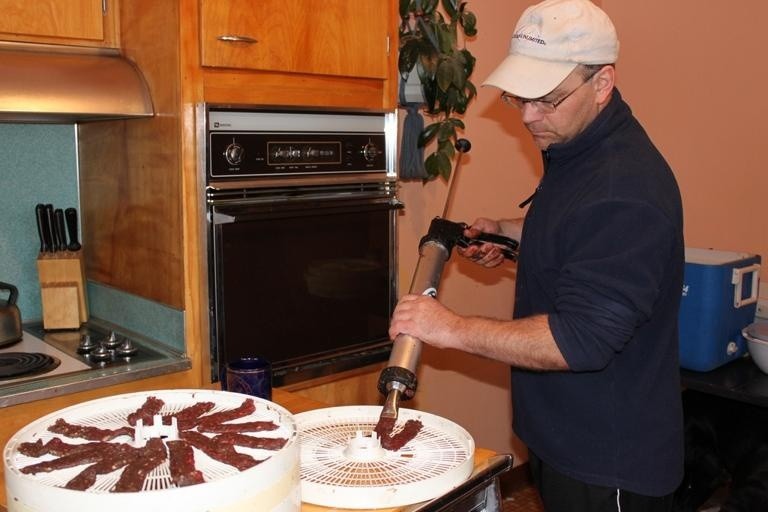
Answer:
[677,246,763,376]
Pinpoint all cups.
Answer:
[219,356,272,401]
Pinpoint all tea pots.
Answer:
[0,281,23,348]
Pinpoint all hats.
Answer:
[481,0,620,99]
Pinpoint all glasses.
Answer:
[500,68,601,114]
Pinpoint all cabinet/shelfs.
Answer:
[74,0,402,115]
[0,0,121,52]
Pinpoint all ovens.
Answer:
[204,109,402,383]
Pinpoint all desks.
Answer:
[668,349,768,508]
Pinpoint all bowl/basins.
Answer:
[742,321,768,374]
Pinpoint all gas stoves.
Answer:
[0,282,158,390]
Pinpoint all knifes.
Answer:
[32,201,81,254]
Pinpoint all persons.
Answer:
[387,1,688,511]
